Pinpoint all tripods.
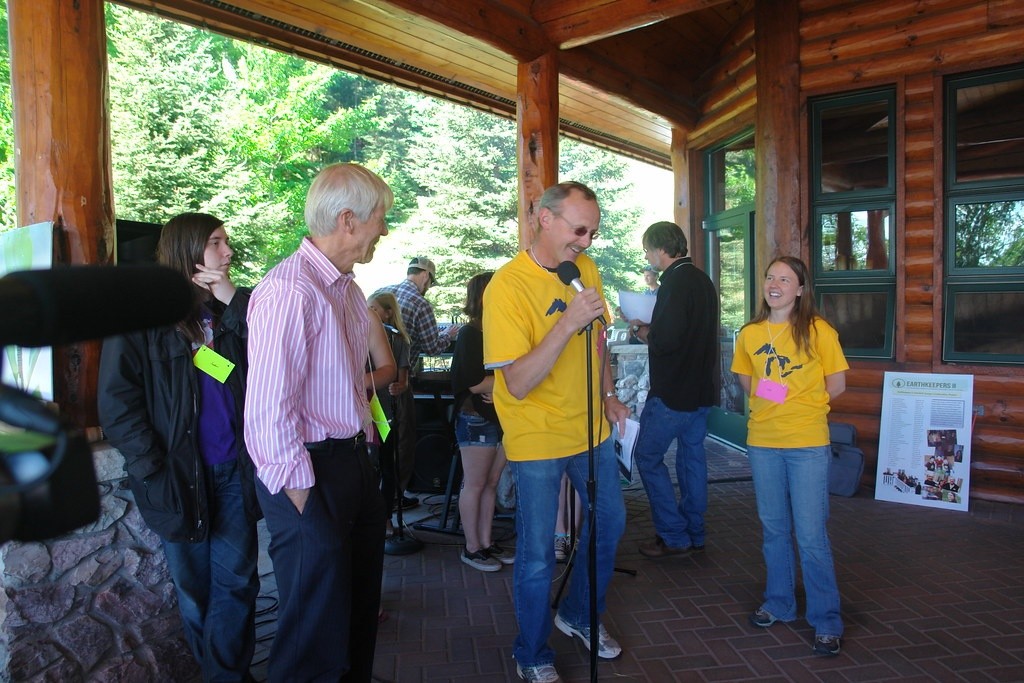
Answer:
[548,484,636,612]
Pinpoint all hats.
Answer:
[409,257,439,288]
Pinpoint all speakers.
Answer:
[406,397,467,497]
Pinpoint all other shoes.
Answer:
[392,496,420,511]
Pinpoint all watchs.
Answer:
[632,324,649,338]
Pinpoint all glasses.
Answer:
[546,207,601,240]
[353,214,389,231]
[643,245,653,253]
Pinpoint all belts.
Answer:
[304,432,367,453]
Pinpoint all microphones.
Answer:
[383,325,400,335]
[0,264,194,347]
[558,260,608,326]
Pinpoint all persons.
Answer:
[455,272,514,572]
[375,256,459,511]
[97,213,250,682]
[637,266,661,296]
[483,180,632,683]
[730,255,850,655]
[883,432,964,504]
[367,291,409,539]
[616,221,721,558]
[243,163,394,683]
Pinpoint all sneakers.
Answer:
[553,534,567,560]
[566,535,580,552]
[638,532,690,559]
[748,607,791,626]
[516,660,563,683]
[484,542,516,565]
[554,613,623,659]
[461,548,502,572]
[690,544,706,553]
[812,633,841,654]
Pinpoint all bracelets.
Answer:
[603,392,618,399]
